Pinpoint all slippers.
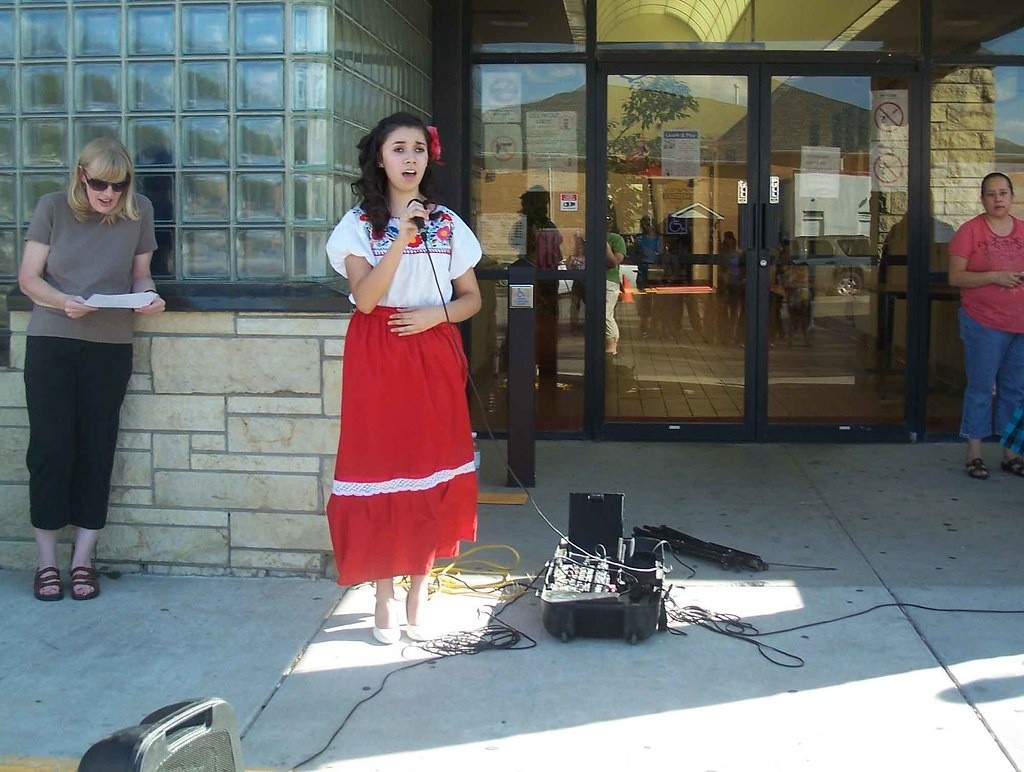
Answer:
[966,457,989,479]
[1000,455,1024,479]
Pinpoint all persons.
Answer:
[566,213,628,360]
[945,173,1024,479]
[325,111,483,643]
[872,189,957,433]
[499,184,564,420]
[17,137,203,600]
[631,201,833,352]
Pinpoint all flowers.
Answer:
[424,126,448,170]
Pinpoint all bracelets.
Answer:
[145,289,162,294]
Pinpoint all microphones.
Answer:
[406,199,427,242]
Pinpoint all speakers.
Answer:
[569,492,624,565]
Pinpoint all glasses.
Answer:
[81,167,129,192]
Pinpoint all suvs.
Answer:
[789,235,872,297]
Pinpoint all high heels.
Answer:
[405,591,429,642]
[373,591,401,645]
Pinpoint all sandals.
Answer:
[70,561,101,600]
[32,565,64,601]
